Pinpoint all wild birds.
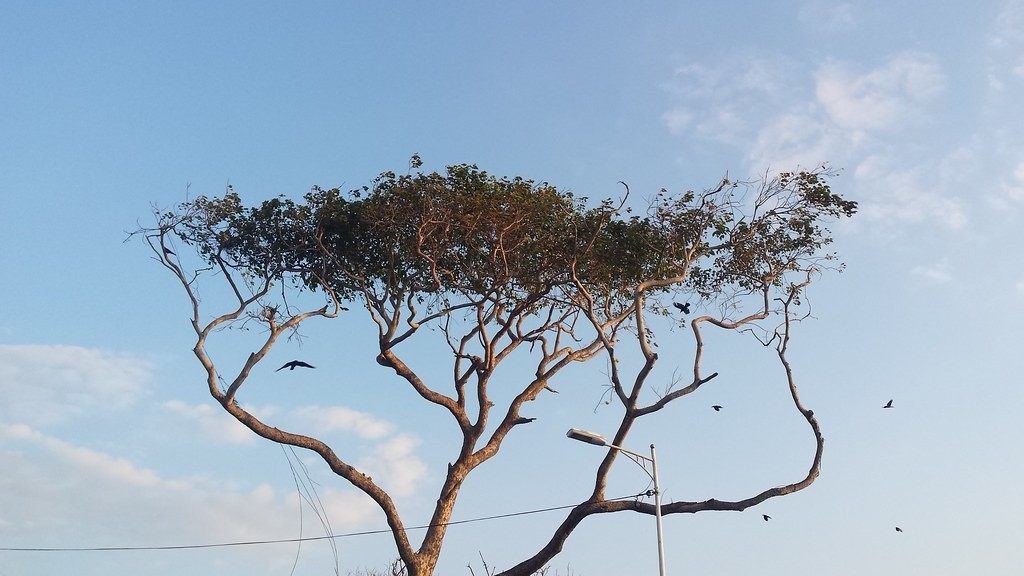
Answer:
[762,514,772,521]
[673,302,691,315]
[712,406,723,412]
[275,360,315,372]
[882,400,894,408]
[165,247,175,254]
[896,527,903,533]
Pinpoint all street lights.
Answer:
[564,427,666,576]
